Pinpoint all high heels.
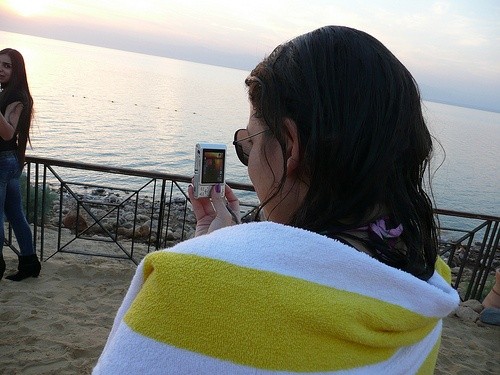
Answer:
[0,254,8,280]
[6,255,42,281]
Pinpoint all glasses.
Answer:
[232,126,274,165]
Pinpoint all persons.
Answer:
[92,26,460,375]
[0,48,41,281]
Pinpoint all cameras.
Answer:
[193,143,227,200]
